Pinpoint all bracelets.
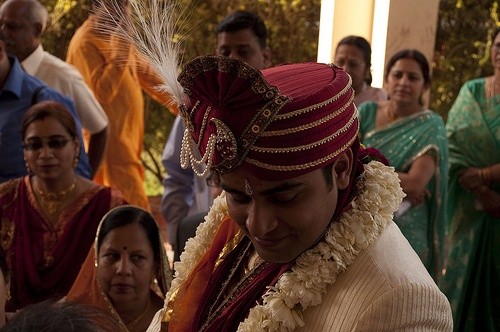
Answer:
[478,166,495,185]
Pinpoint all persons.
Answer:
[66,1,181,213]
[0,244,17,332]
[438,27,499,331]
[160,10,269,273]
[0,100,131,324]
[333,35,389,108]
[145,64,454,332]
[356,48,448,297]
[52,204,173,332]
[0,30,92,184]
[0,0,110,182]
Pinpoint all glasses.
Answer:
[21,134,74,149]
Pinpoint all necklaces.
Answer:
[34,175,78,214]
[125,297,152,328]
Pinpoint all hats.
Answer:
[94,0,360,182]
